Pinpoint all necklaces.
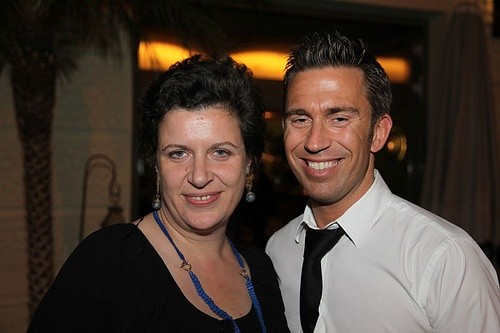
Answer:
[152,211,266,332]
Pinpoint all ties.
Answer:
[298,222,343,333]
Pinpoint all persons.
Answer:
[264,38,500,333]
[24,53,293,333]
